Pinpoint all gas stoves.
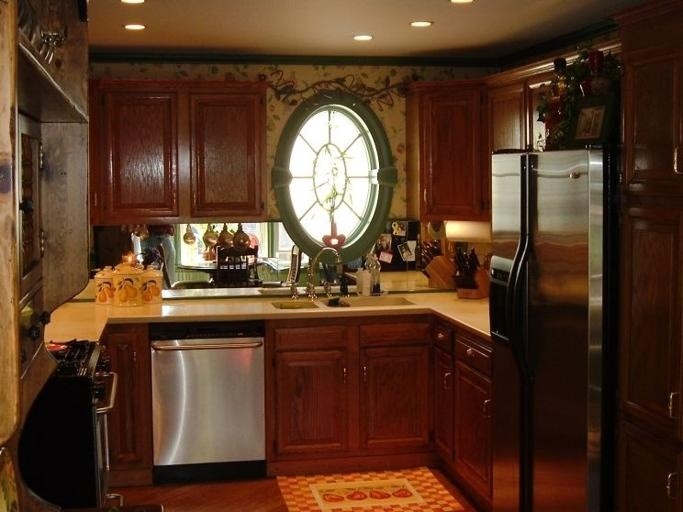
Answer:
[44,338,101,380]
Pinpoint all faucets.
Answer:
[306,246,344,299]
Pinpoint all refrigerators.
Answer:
[488,142,614,512]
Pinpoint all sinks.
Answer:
[321,294,417,308]
[270,300,320,308]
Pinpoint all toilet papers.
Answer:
[445,221,492,243]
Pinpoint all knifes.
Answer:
[415,239,442,278]
[452,245,481,276]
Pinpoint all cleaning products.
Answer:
[368,254,381,296]
[356,268,371,297]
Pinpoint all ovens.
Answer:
[141,319,269,486]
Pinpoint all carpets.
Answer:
[276,466,467,512]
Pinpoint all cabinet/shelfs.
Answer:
[617,53,682,512]
[17,0,91,123]
[488,79,557,154]
[99,86,265,219]
[421,91,485,214]
[18,110,49,376]
[107,332,145,469]
[432,325,451,466]
[455,334,493,506]
[273,323,434,461]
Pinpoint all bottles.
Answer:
[94,262,164,308]
[363,252,381,296]
[544,58,577,151]
